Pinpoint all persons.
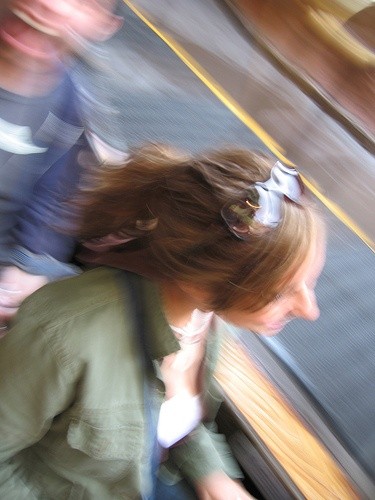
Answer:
[1,143,329,500]
[1,2,126,326]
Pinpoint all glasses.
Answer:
[222,158,310,243]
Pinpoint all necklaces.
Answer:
[167,324,210,343]
